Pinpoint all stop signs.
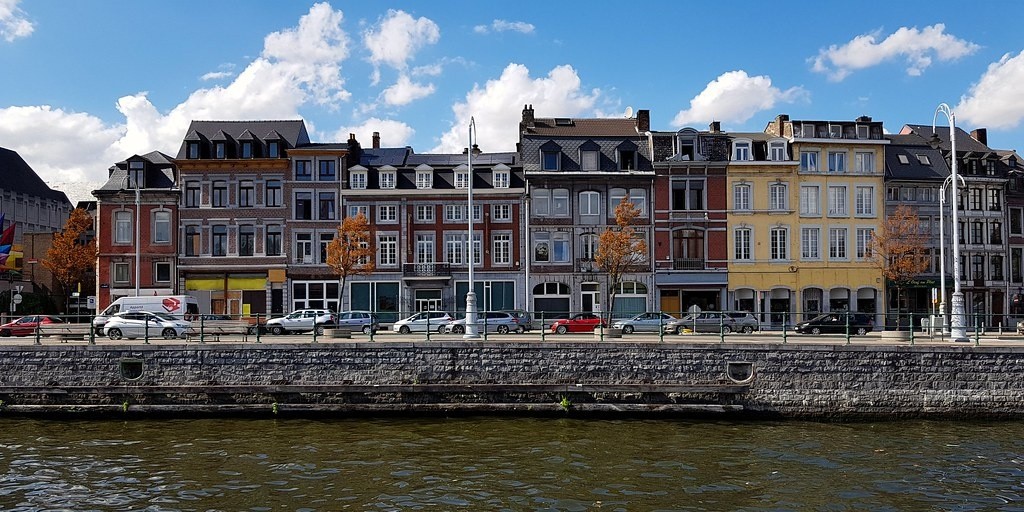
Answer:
[1014,296,1019,303]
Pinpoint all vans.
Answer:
[93,296,199,337]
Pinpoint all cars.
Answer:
[503,309,531,334]
[248,315,301,335]
[794,311,873,336]
[0,316,65,337]
[552,313,607,335]
[103,310,192,340]
[723,310,758,334]
[613,312,677,334]
[393,311,455,335]
[444,311,518,335]
[198,314,232,334]
[663,311,737,334]
[335,311,380,335]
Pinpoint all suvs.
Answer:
[266,308,336,335]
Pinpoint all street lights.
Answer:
[116,176,141,297]
[925,102,971,342]
[463,116,484,337]
[938,173,971,337]
